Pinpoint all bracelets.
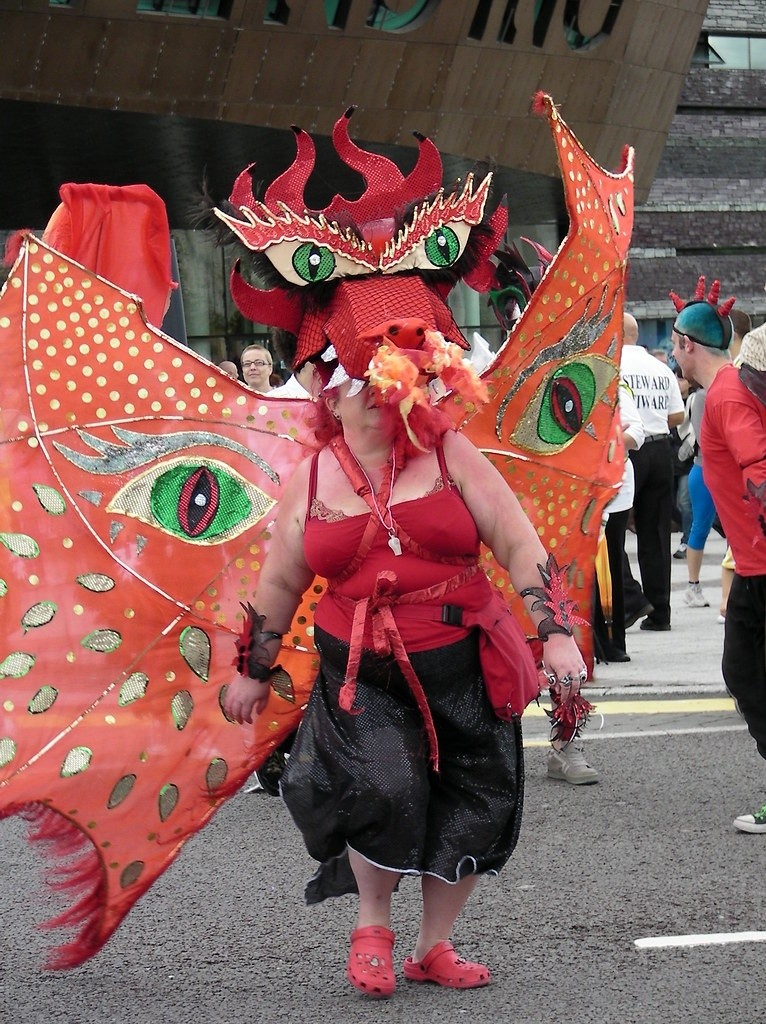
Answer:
[230,601,283,684]
[519,553,592,645]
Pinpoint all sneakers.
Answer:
[733,805,766,833]
[682,584,710,607]
[546,749,599,784]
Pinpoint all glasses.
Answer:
[241,359,271,367]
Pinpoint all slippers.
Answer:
[347,925,395,997]
[403,941,491,988]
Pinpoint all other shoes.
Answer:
[594,647,631,662]
[624,596,654,629]
[673,548,687,559]
[640,617,671,631]
[717,614,725,624]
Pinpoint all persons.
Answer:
[224,358,589,997]
[488,276,766,834]
[217,325,321,400]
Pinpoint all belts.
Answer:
[644,434,668,442]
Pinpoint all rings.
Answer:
[559,674,572,688]
[544,672,556,686]
[571,675,580,682]
[580,668,588,683]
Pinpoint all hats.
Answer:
[668,275,736,350]
